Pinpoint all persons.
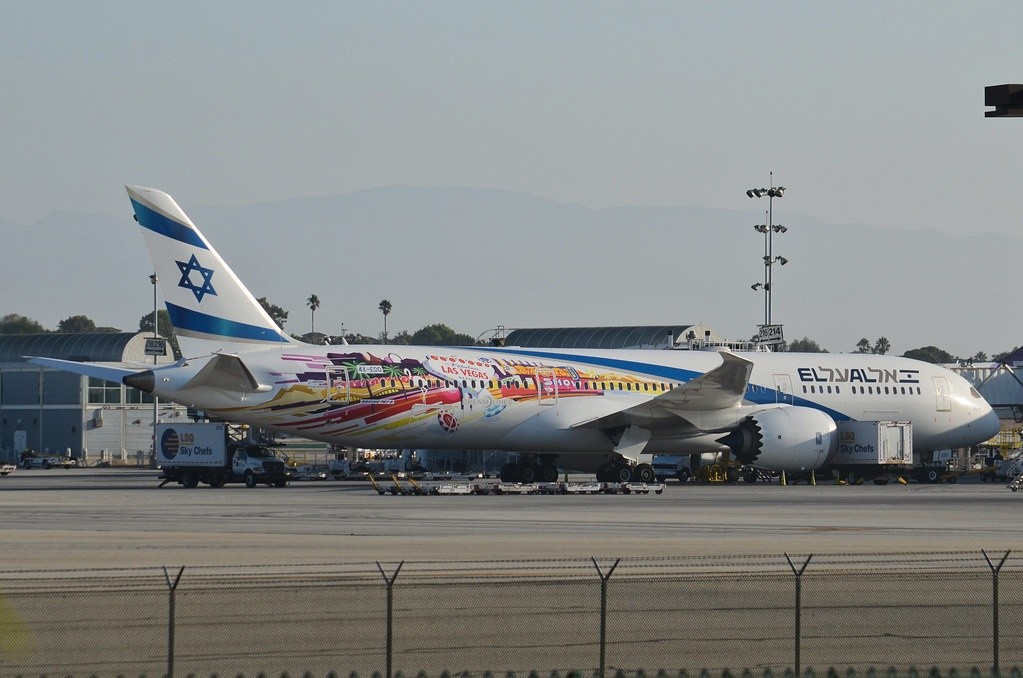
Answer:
[993,449,1003,461]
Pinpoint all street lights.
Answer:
[148,273,158,469]
[745,173,786,324]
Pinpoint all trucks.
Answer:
[652,453,714,482]
[156,421,284,488]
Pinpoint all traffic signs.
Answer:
[755,325,783,344]
[144,336,166,356]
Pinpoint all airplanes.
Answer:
[20,186,1001,485]
